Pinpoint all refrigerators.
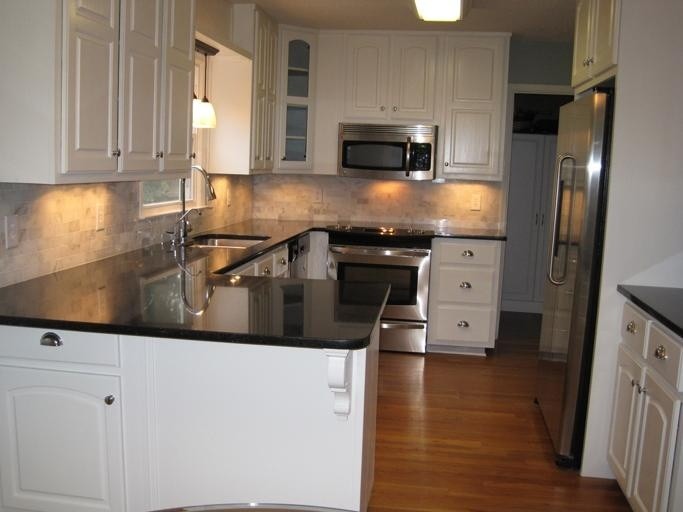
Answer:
[534,92,611,460]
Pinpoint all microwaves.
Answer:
[337,121,438,182]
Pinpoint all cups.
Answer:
[160,232,175,251]
[434,218,449,235]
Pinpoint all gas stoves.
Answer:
[326,223,436,250]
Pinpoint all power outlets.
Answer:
[470,191,482,210]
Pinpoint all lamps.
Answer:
[414,0,463,23]
[194,39,219,128]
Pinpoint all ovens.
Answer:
[327,244,430,354]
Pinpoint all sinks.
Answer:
[191,234,274,250]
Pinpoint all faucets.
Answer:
[179,165,217,243]
[170,207,201,253]
[171,248,201,278]
[179,244,216,316]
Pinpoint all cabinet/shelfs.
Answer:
[217,232,329,279]
[607,297,683,512]
[570,0,621,96]
[160,0,196,180]
[276,23,512,183]
[209,0,274,177]
[0,324,128,511]
[425,235,506,357]
[0,0,159,185]
